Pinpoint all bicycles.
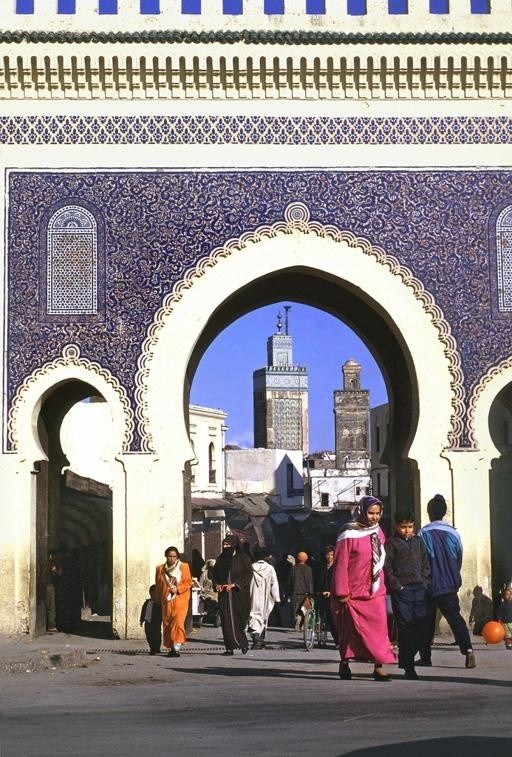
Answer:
[296,591,326,651]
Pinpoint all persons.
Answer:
[416,494,476,667]
[498,589,512,650]
[331,496,399,681]
[139,535,343,656]
[384,507,432,680]
[47,549,86,633]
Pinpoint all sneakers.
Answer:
[505,644,511,649]
[173,650,181,656]
[250,640,266,649]
[149,650,156,655]
[242,646,248,654]
[156,649,162,652]
[414,657,433,666]
[402,667,419,678]
[168,650,174,656]
[223,650,234,655]
[464,652,476,668]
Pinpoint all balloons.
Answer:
[482,622,506,645]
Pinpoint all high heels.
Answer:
[337,662,352,680]
[371,668,395,681]
